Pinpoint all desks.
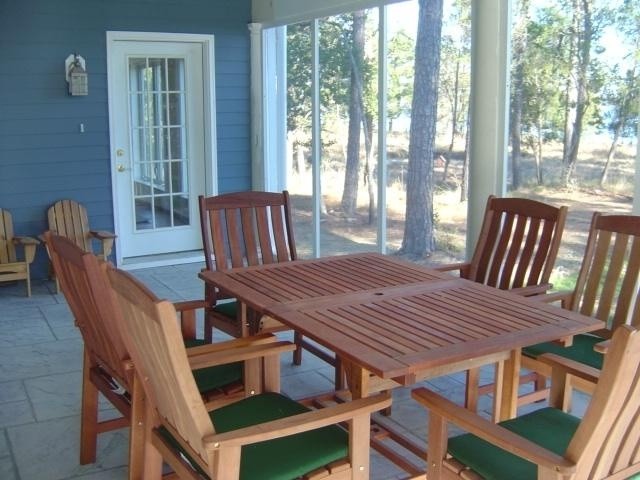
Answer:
[196,251,606,479]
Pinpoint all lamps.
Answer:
[66,53,89,97]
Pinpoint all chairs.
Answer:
[0,198,119,297]
[429,193,570,412]
[498,211,640,412]
[44,228,277,480]
[410,322,640,480]
[197,189,305,366]
[100,258,393,480]
